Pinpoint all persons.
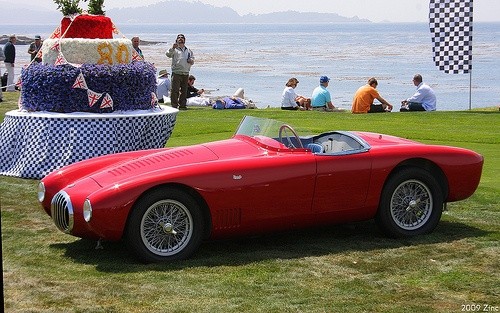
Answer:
[280,78,308,111]
[399,74,437,112]
[1,71,9,92]
[131,36,145,63]
[27,34,44,61]
[166,33,195,110]
[310,75,337,111]
[176,74,205,108]
[351,77,394,113]
[154,69,173,103]
[212,87,256,109]
[3,33,17,91]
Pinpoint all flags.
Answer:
[429,0,474,76]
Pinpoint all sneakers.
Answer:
[179,106,189,110]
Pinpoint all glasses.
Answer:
[178,36,184,38]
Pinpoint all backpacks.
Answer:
[1,72,8,91]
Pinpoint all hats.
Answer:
[35,33,40,38]
[9,33,16,36]
[216,99,224,108]
[158,68,170,77]
[320,76,330,82]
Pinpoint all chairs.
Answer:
[304,143,324,153]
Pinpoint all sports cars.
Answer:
[37,115,485,261]
